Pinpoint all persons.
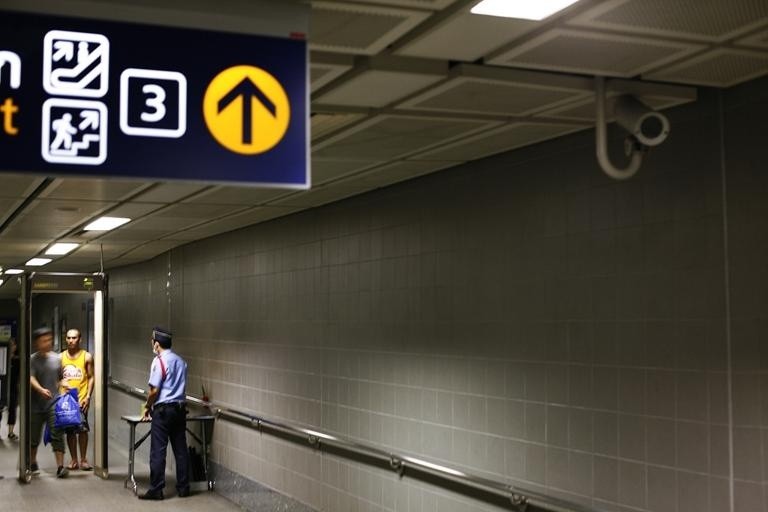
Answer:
[57,328,94,470]
[137,326,190,499]
[0,337,20,445]
[28,326,72,479]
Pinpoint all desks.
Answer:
[120,414,217,497]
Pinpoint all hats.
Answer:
[151,326,173,343]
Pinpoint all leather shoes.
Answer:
[179,487,191,497]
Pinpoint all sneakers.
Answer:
[138,486,165,500]
[67,460,93,470]
[30,463,40,475]
[56,467,69,477]
[8,433,19,440]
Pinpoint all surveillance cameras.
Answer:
[613,93,670,146]
